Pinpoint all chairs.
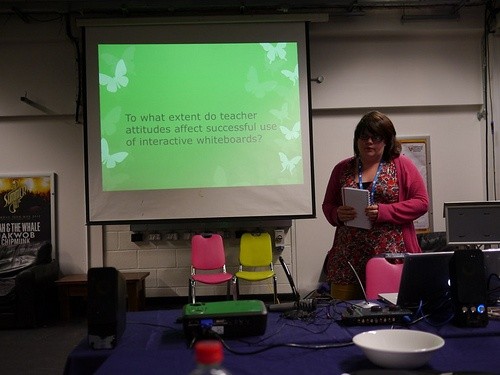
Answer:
[190,233,233,304]
[360,253,405,300]
[233,233,277,305]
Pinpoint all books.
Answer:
[340,187,372,229]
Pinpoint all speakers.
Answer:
[86,266,126,350]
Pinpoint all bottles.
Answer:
[189,342,231,375]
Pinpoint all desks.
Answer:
[52,272,149,311]
[64,300,500,375]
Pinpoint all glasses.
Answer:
[360,133,385,142]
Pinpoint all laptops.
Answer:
[377,248,500,308]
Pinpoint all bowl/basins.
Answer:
[353,330,445,366]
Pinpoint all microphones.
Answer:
[268,298,320,311]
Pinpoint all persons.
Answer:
[322,111,430,301]
